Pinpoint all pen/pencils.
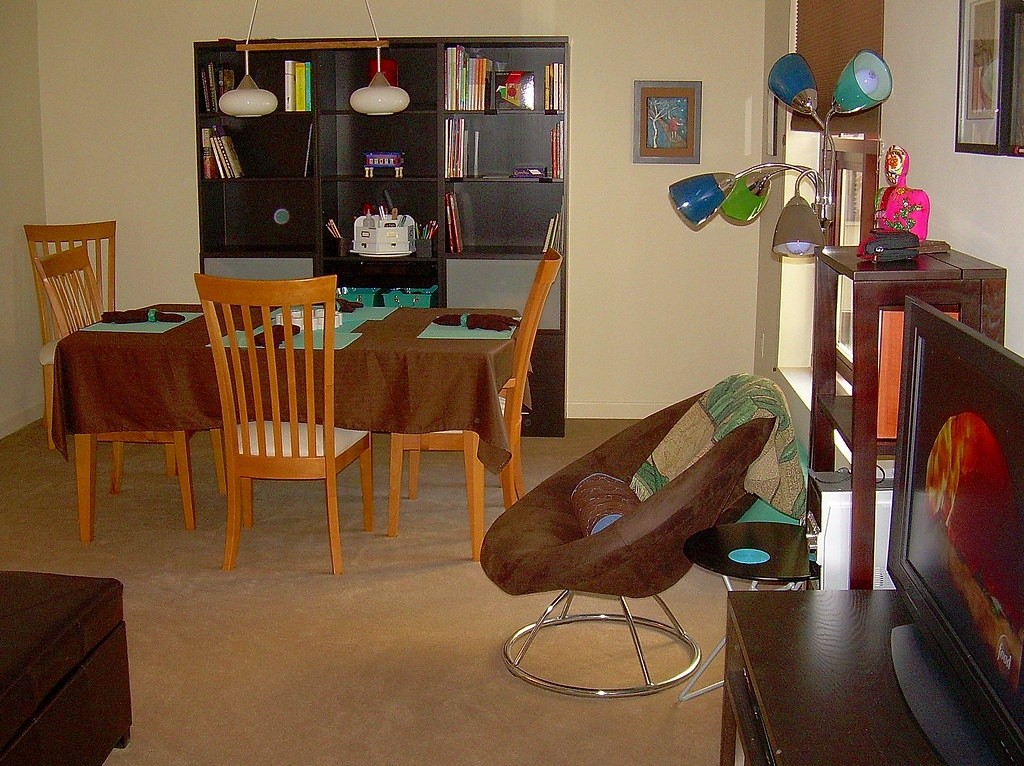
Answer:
[377,205,387,220]
[325,219,343,238]
[397,215,407,227]
[416,221,438,239]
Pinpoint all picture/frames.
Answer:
[633,80,703,164]
[954,0,1024,158]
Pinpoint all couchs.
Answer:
[0,570,132,766]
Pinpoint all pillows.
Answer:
[570,473,642,539]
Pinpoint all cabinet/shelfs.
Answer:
[805,245,1008,591]
[193,35,570,437]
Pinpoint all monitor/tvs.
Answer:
[885,294,1024,766]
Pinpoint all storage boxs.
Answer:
[337,288,382,307]
[381,285,438,308]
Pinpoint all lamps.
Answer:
[218,0,411,117]
[668,49,893,259]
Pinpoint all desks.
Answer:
[678,522,809,702]
[719,590,949,766]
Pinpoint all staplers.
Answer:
[865,236,919,263]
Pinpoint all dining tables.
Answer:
[51,302,534,561]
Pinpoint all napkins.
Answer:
[299,299,364,313]
[254,325,300,349]
[101,308,186,324]
[432,313,520,332]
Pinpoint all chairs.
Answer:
[23,221,116,450]
[386,247,564,538]
[193,272,373,576]
[35,245,229,530]
[480,374,808,697]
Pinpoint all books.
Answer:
[303,122,314,178]
[199,126,244,179]
[540,210,558,253]
[549,119,566,184]
[444,190,464,254]
[443,44,565,113]
[284,58,314,112]
[199,57,236,114]
[443,116,480,182]
